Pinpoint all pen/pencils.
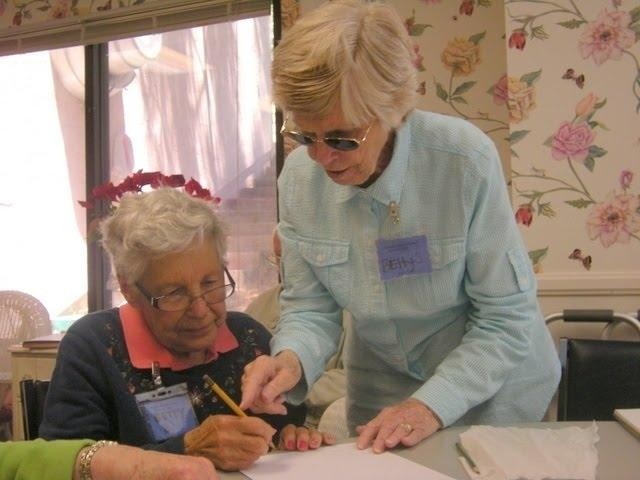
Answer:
[203,372,276,449]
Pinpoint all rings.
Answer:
[400,422,413,431]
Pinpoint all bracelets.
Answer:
[79,440,118,480]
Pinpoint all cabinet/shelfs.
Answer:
[7,330,63,439]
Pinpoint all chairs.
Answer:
[541,306,639,423]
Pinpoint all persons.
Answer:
[39,189,336,472]
[239,1,561,455]
[0,438,217,480]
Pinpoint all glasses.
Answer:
[134,267,236,312]
[279,114,375,152]
